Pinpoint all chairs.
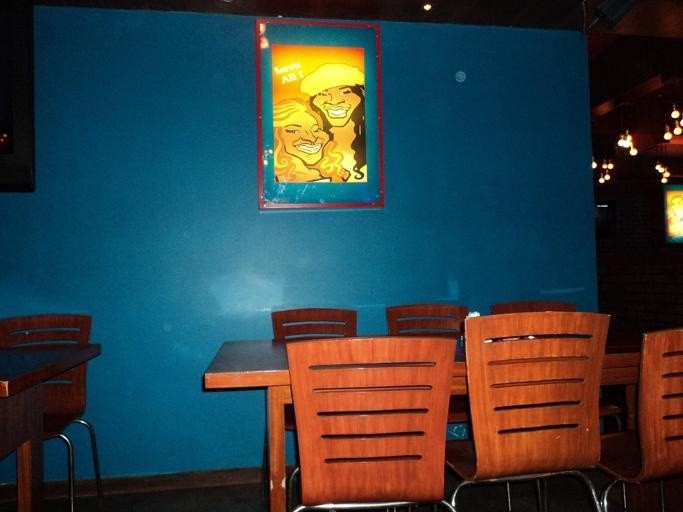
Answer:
[1,313,105,511]
[542,327,682,511]
[284,336,457,511]
[385,303,470,426]
[446,311,612,512]
[490,301,621,431]
[260,308,357,495]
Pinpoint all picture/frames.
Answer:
[254,18,385,210]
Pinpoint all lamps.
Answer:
[589,103,682,187]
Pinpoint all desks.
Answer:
[203,333,641,510]
[2,343,100,509]
